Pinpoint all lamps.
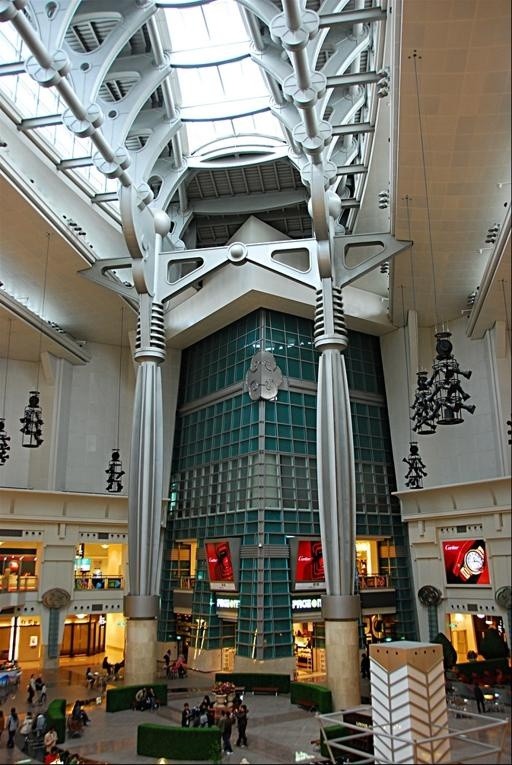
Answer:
[105,307,126,492]
[401,50,475,491]
[20,233,51,448]
[0,319,12,466]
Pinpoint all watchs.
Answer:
[457,545,485,583]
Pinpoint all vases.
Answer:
[215,694,227,705]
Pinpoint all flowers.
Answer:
[209,681,235,695]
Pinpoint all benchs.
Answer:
[231,687,245,695]
[131,699,160,711]
[251,687,279,696]
[68,713,83,733]
[298,699,319,712]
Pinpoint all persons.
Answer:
[86,656,125,693]
[360,653,369,681]
[135,687,159,713]
[473,683,487,715]
[176,654,186,679]
[71,700,92,727]
[0,660,58,765]
[163,649,173,680]
[181,694,249,756]
